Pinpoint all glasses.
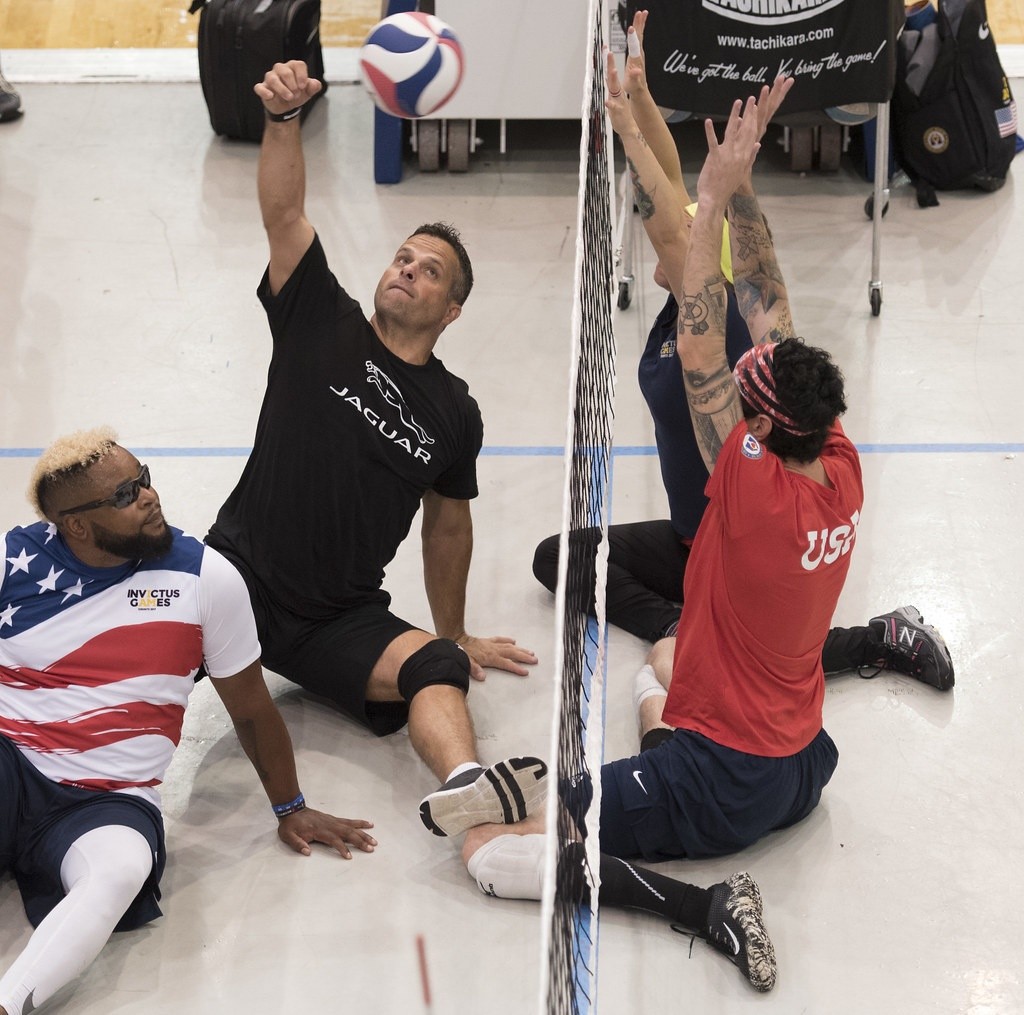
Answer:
[58,465,151,517]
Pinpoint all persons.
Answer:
[465,336,865,995]
[533,6,959,696]
[1,421,379,1014]
[198,58,552,840]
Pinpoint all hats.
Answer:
[684,199,734,284]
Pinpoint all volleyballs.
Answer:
[356,8,467,122]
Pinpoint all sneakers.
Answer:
[417,755,548,836]
[670,872,777,994]
[857,604,954,691]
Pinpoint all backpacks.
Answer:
[185,0,327,142]
[877,0,1018,206]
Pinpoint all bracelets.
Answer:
[271,792,306,818]
[264,107,304,123]
